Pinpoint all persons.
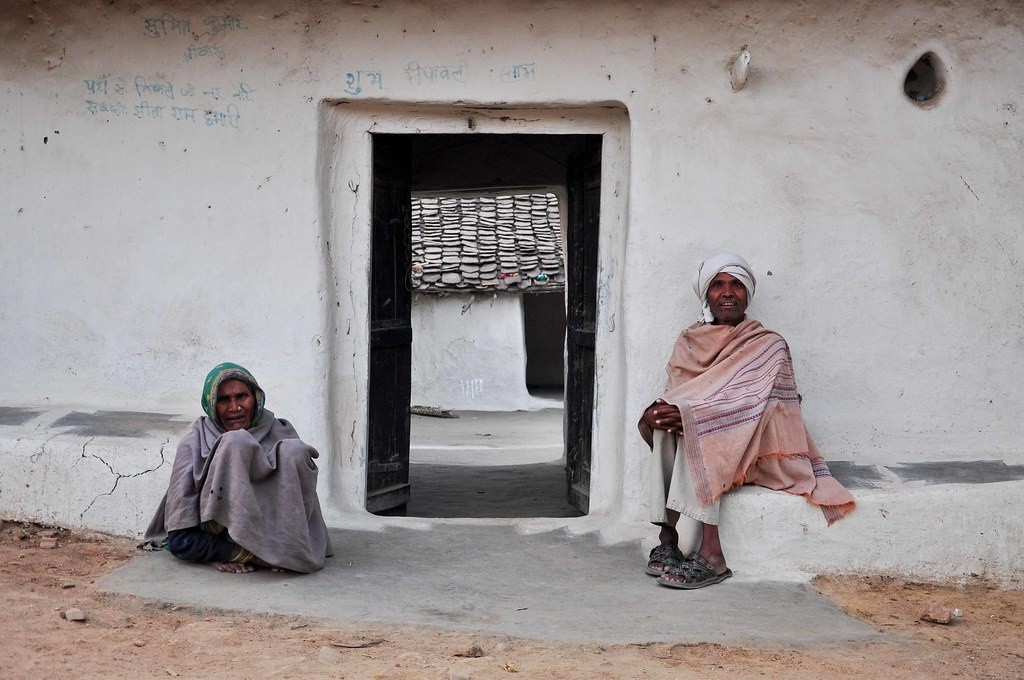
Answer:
[144,361,330,573]
[635,253,856,590]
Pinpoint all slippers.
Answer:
[646,544,684,576]
[657,553,733,588]
